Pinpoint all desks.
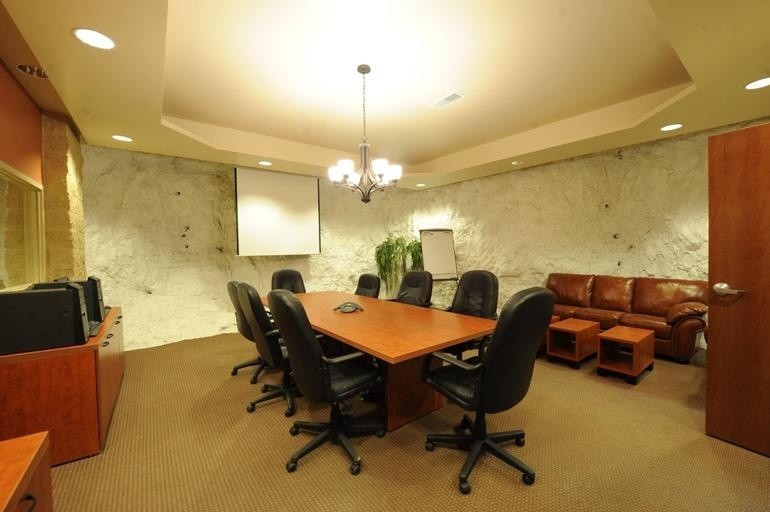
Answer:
[259,289,498,432]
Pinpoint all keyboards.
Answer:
[88,320,101,336]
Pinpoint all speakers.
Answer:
[0,280,89,355]
[33,275,105,322]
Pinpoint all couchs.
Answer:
[544,272,710,365]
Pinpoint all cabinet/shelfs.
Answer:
[1,303,126,468]
[536,315,561,358]
[0,428,57,512]
[546,315,600,369]
[594,323,656,383]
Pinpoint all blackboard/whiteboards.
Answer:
[419,229,459,281]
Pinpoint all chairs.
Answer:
[225,279,280,385]
[352,272,379,298]
[440,269,499,360]
[267,288,387,475]
[388,269,433,306]
[268,268,306,296]
[238,283,324,418]
[420,286,556,495]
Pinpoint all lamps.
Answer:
[327,65,405,206]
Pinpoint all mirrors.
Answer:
[0,160,46,297]
